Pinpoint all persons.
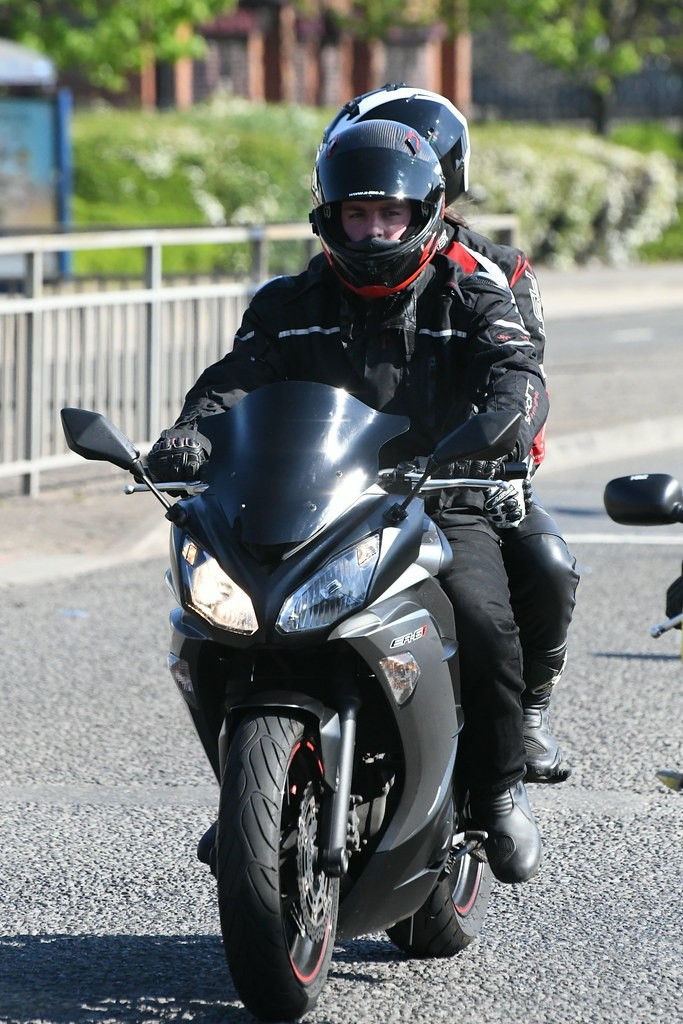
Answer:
[147,116,547,889]
[310,81,577,784]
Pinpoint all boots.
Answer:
[520,640,571,785]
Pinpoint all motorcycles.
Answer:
[58,403,495,1015]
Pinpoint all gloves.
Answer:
[147,426,211,480]
[431,453,507,492]
[487,478,526,529]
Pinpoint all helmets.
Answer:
[306,119,447,299]
[334,83,470,209]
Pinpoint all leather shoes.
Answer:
[471,775,543,883]
